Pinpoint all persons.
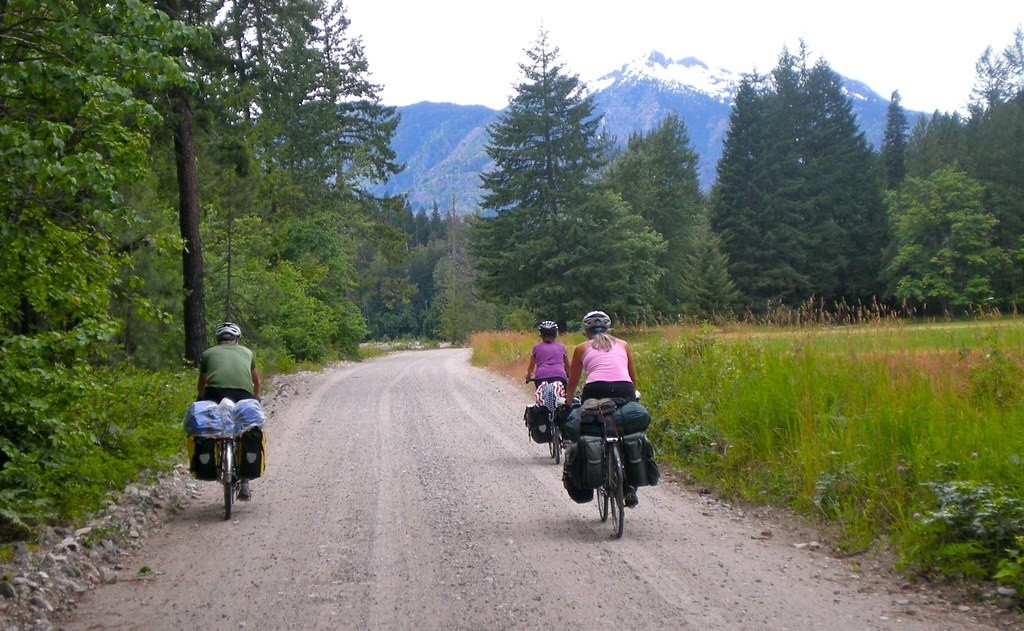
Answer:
[562,310,644,511]
[525,320,574,449]
[197,322,260,501]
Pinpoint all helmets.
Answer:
[538,321,558,335]
[582,311,611,330]
[215,322,241,339]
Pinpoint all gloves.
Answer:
[252,396,261,403]
[558,397,572,408]
[635,389,641,399]
[195,395,203,401]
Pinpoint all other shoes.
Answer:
[239,488,252,501]
[563,439,572,449]
[625,490,638,506]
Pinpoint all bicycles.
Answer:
[568,398,627,538]
[526,377,566,463]
[194,394,259,519]
